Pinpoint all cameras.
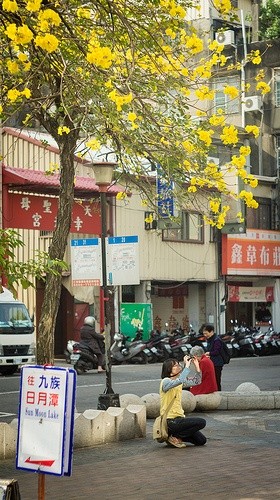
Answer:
[188,357,194,361]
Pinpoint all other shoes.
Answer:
[168,433,186,448]
[98,369,106,373]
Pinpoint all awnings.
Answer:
[2,167,132,197]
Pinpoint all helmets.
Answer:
[83,316,95,327]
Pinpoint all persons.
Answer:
[159,355,207,448]
[203,324,224,391]
[189,346,217,395]
[81,316,106,372]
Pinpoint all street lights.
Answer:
[83,159,121,411]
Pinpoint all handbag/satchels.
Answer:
[152,389,179,443]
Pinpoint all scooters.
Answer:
[107,333,150,365]
[71,336,112,375]
[134,319,280,361]
[64,339,79,364]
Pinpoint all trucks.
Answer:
[0,288,37,371]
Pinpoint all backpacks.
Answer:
[212,338,232,364]
